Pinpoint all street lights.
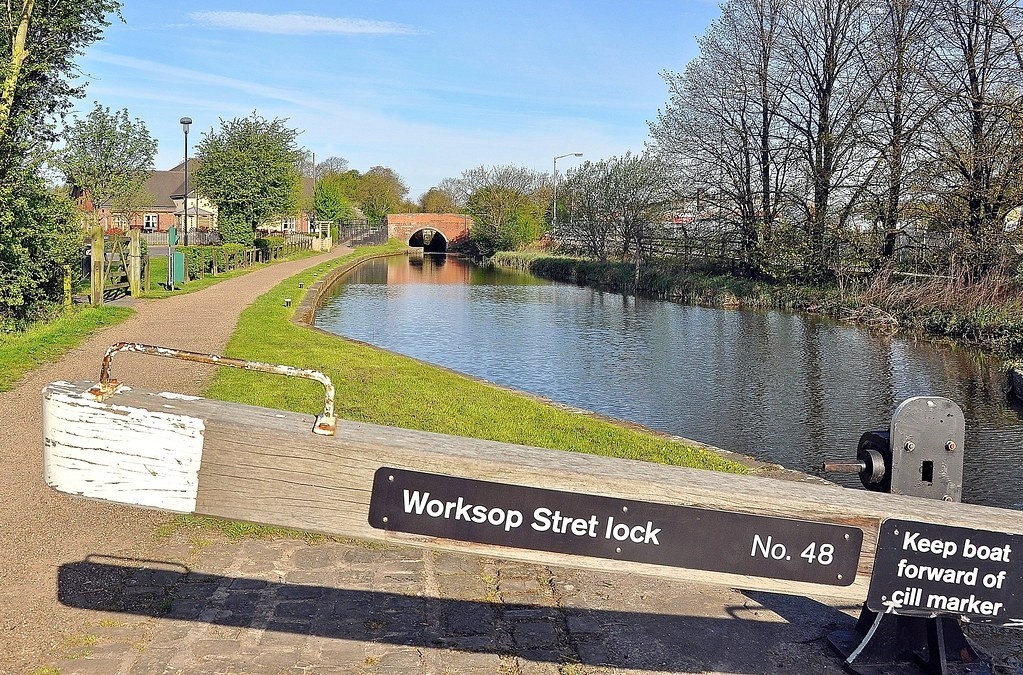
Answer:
[180,116,192,245]
[553,152,583,230]
